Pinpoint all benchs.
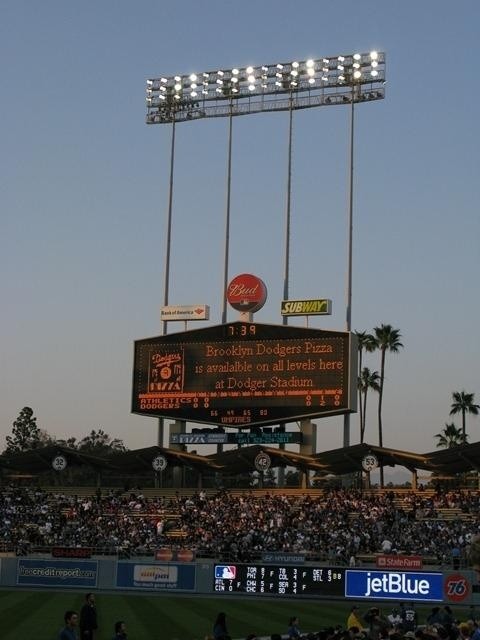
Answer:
[0,485,479,539]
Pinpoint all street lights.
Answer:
[143,47,390,456]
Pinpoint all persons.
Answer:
[109,621,129,639]
[213,611,229,638]
[2,468,480,571]
[203,623,346,640]
[287,615,302,637]
[57,609,80,639]
[78,593,98,639]
[347,601,480,639]
